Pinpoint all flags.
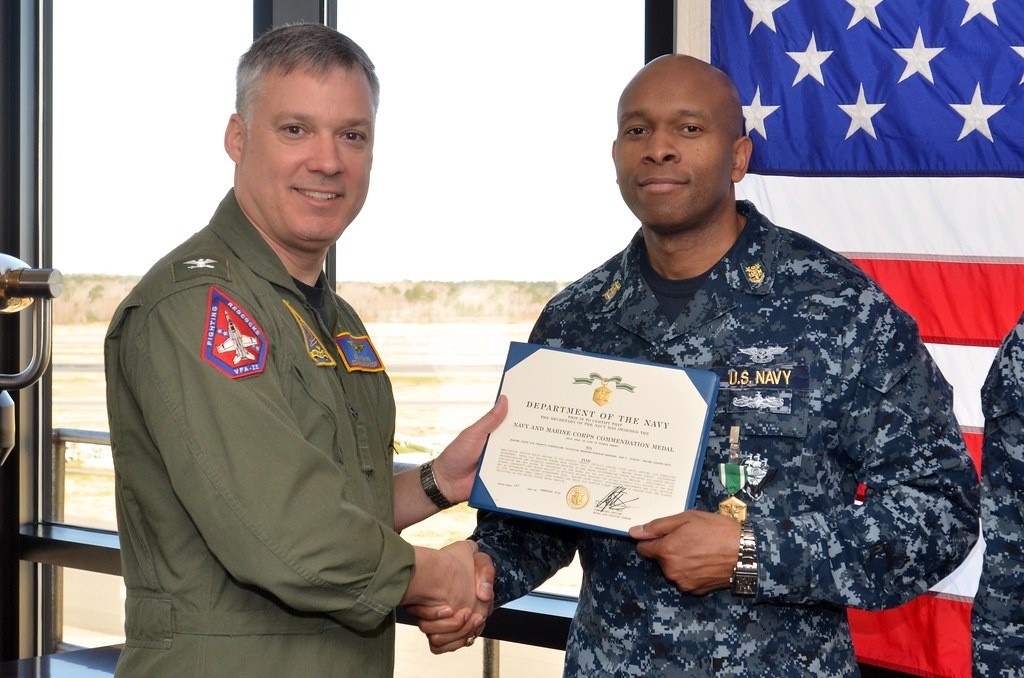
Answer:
[709,1,1023,677]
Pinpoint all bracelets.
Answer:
[419,460,454,511]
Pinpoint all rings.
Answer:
[467,637,473,646]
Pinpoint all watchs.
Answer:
[729,520,757,596]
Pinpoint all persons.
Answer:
[971,308,1024,678]
[103,21,508,678]
[400,53,984,678]
